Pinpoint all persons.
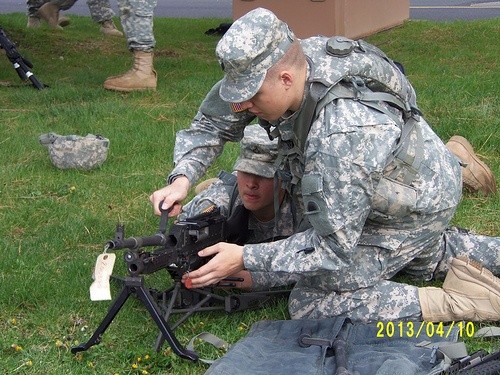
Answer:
[26,0,123,37]
[103,0,158,91]
[149,8,500,324]
[173,124,496,290]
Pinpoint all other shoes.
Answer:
[27,16,41,28]
[56,13,70,25]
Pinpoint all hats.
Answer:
[214,7,294,103]
[233,125,285,178]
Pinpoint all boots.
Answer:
[418,255,500,323]
[103,50,158,91]
[38,2,63,31]
[100,19,124,36]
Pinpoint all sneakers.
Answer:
[446,136,495,197]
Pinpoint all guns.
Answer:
[71,200,255,361]
